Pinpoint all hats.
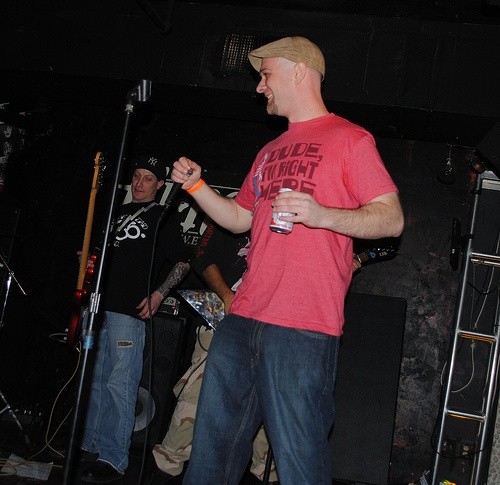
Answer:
[134,155,167,179]
[248,36,326,82]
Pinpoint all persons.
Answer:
[147,191,362,485]
[180,37,404,485]
[50,157,192,485]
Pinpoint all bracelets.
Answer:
[187,179,205,195]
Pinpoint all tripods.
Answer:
[0,256,32,445]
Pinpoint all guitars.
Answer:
[67,151,107,345]
[175,246,394,332]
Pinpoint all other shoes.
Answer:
[145,469,176,485]
[78,452,125,483]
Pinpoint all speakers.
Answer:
[328,292,407,485]
[128,312,190,453]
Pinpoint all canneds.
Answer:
[270,188,295,234]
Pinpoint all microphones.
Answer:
[159,168,192,218]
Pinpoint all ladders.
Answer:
[429,173,500,485]
